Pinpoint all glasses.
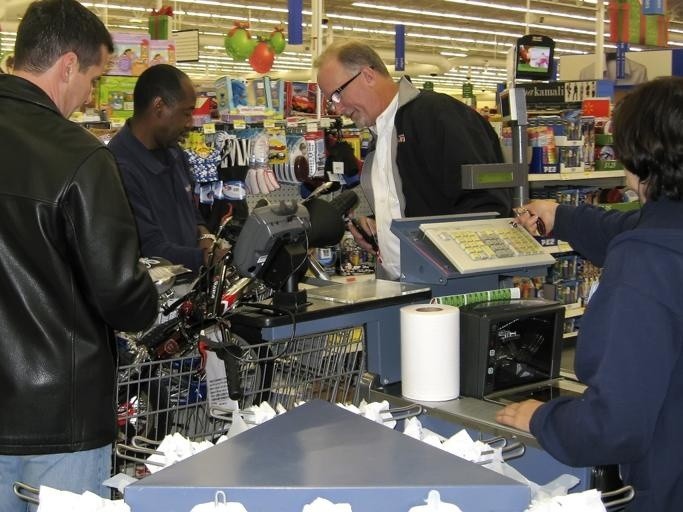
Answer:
[325,64,376,107]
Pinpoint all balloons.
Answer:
[248,34,275,75]
[268,27,287,54]
[223,20,253,64]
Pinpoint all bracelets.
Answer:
[197,232,217,243]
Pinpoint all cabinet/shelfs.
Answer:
[491,116,628,341]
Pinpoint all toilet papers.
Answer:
[400,304,462,401]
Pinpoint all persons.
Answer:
[495,73,683,511]
[0,0,160,512]
[108,63,233,299]
[311,38,515,279]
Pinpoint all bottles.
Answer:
[478,106,512,146]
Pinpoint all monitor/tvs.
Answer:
[517,42,551,76]
